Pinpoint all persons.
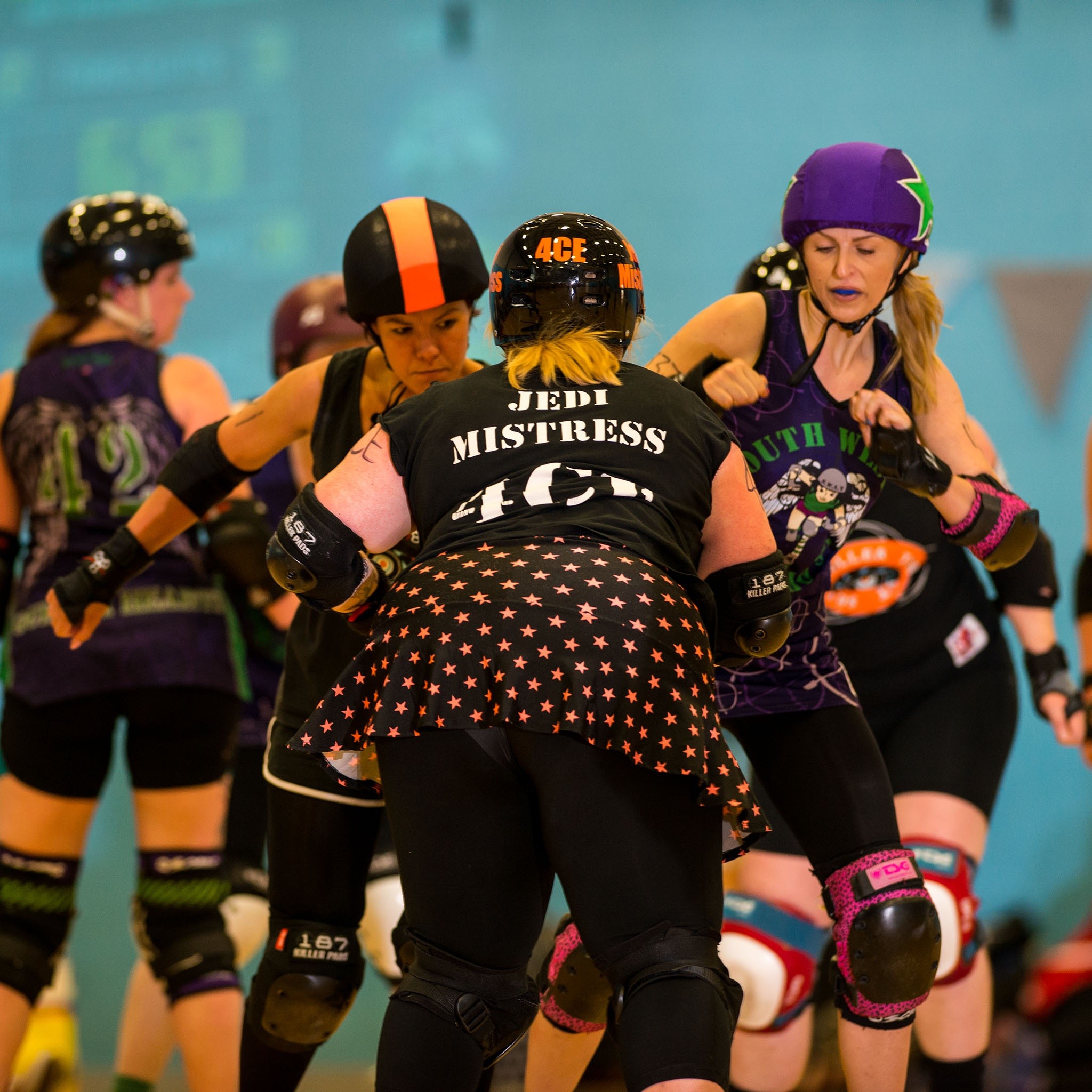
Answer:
[0,142,1092,1092]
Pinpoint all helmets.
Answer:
[782,142,933,253]
[42,191,193,300]
[344,196,489,324]
[736,244,805,293]
[275,277,368,348]
[490,208,648,345]
